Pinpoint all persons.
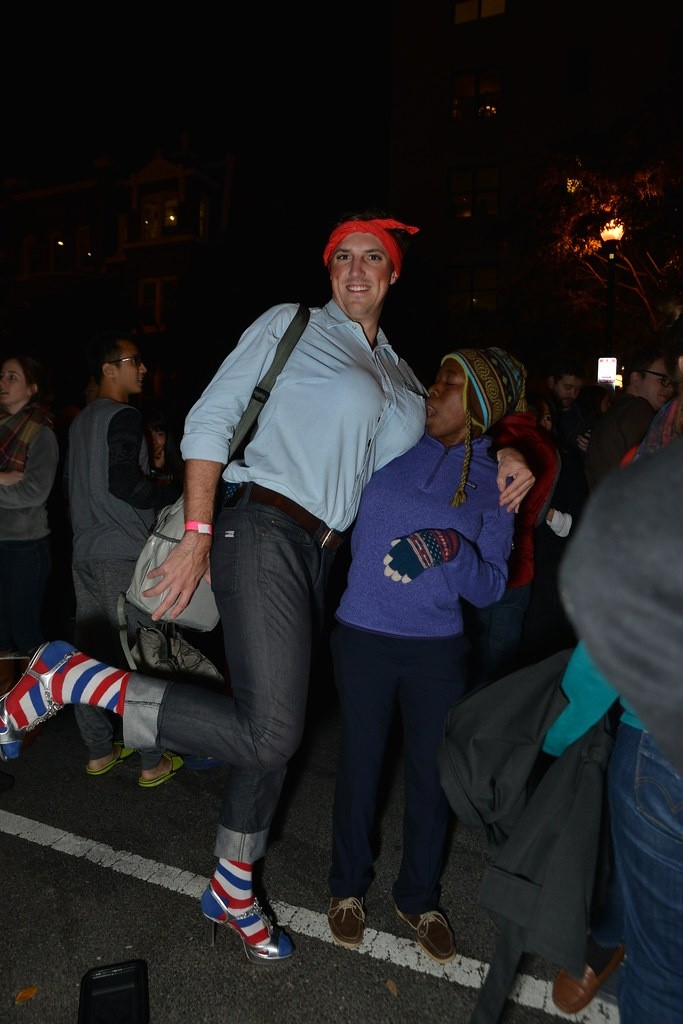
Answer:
[478,313,683,1024]
[69,334,184,787]
[0,209,536,958]
[329,346,526,961]
[0,357,59,750]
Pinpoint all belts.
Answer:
[237,480,342,553]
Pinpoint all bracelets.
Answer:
[185,520,212,535]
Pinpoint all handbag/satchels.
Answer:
[125,491,221,634]
[122,627,223,690]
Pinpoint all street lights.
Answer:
[597,214,626,387]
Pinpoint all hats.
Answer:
[440,347,527,508]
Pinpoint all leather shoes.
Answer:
[553,940,626,1014]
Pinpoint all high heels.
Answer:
[0,642,80,762]
[199,885,296,964]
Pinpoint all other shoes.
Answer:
[327,894,363,944]
[394,900,455,963]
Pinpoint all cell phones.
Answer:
[78,959,150,1024]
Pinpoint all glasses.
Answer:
[101,353,143,367]
[633,368,672,388]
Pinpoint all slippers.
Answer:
[138,748,184,788]
[85,738,135,776]
